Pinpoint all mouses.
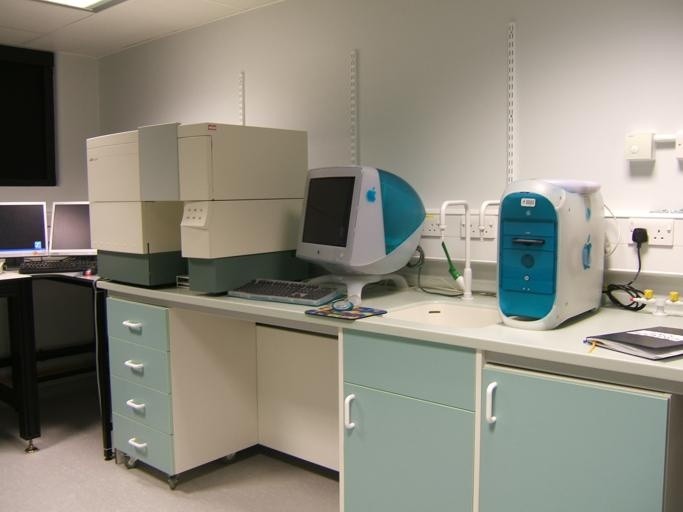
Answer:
[83,268,95,274]
[332,299,353,311]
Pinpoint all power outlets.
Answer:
[629,217,647,246]
[421,219,442,237]
[459,215,481,239]
[481,216,496,239]
[646,219,673,246]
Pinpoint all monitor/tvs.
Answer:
[49,201,97,261]
[296,166,426,306]
[0,202,49,267]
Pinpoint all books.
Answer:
[584,325,683,362]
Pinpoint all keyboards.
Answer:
[228,278,340,306]
[19,261,97,274]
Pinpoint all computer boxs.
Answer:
[496,179,605,330]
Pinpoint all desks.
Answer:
[0,258,113,463]
[93,259,683,512]
